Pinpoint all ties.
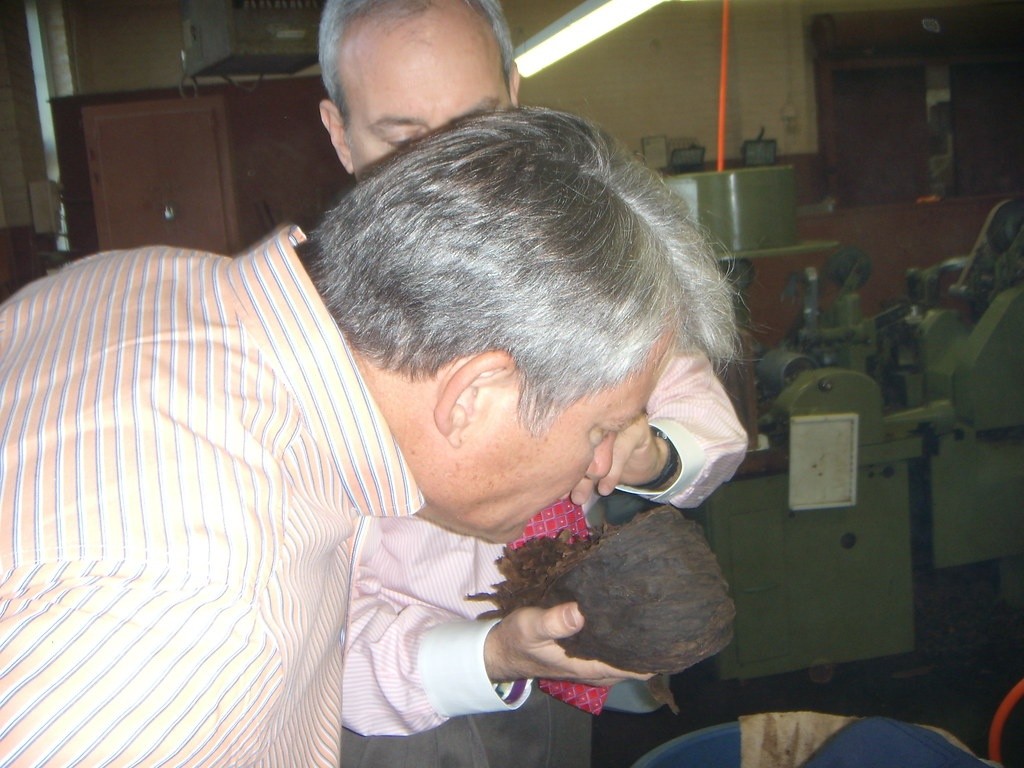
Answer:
[507,494,614,716]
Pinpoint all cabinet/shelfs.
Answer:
[697,461,917,680]
[53,92,244,259]
[816,48,1024,209]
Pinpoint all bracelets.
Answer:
[639,426,678,491]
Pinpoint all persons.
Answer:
[316,0,750,768]
[0,108,740,768]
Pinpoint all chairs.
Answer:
[628,679,1024,768]
